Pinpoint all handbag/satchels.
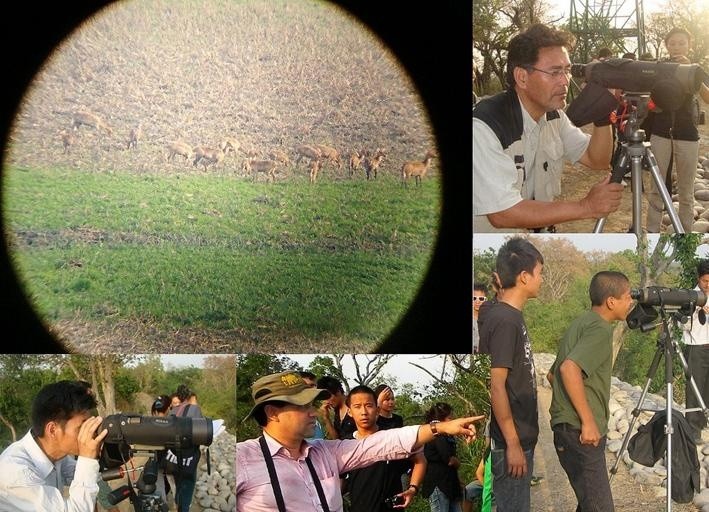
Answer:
[159,448,201,478]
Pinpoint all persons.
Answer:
[0,381,118,511]
[677,260,708,436]
[582,30,708,233]
[471,26,624,233]
[152,394,169,418]
[81,383,92,394]
[301,371,492,511]
[170,394,184,407]
[236,372,484,511]
[547,272,634,511]
[476,276,504,333]
[145,384,204,512]
[474,285,489,352]
[479,239,545,510]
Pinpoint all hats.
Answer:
[241,372,332,422]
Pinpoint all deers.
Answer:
[58,111,435,189]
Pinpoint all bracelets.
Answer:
[428,419,440,436]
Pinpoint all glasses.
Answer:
[532,67,571,78]
[474,297,484,301]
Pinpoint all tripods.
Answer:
[610,305,709,512]
[593,90,686,232]
